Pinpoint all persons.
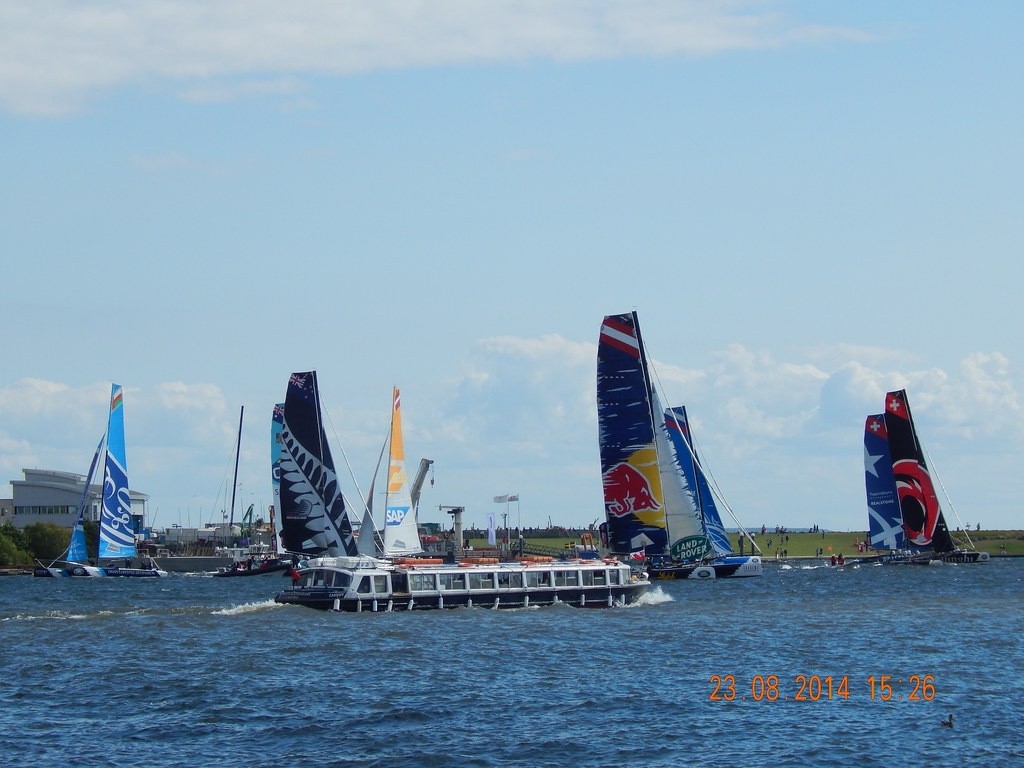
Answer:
[227,553,312,571]
[738,522,922,565]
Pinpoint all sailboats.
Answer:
[32,313,989,586]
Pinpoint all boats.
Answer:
[273,548,653,613]
